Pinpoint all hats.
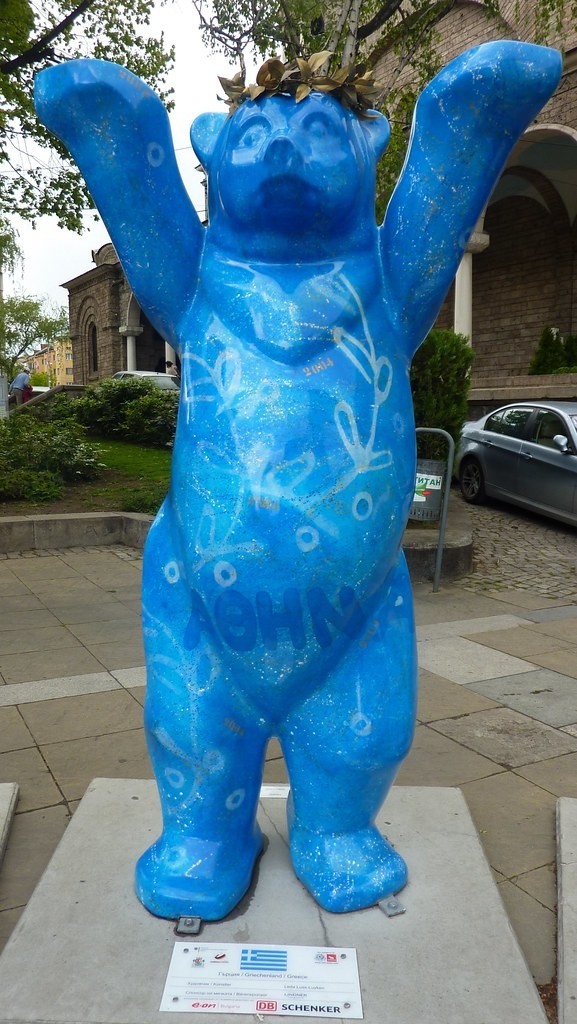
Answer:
[24,367,31,372]
[164,361,173,364]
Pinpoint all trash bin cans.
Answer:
[408,459,446,521]
[23,384,33,403]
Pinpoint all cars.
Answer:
[453,400,577,530]
[112,371,181,393]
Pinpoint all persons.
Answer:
[165,360,181,381]
[7,366,30,406]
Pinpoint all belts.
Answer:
[14,388,23,391]
